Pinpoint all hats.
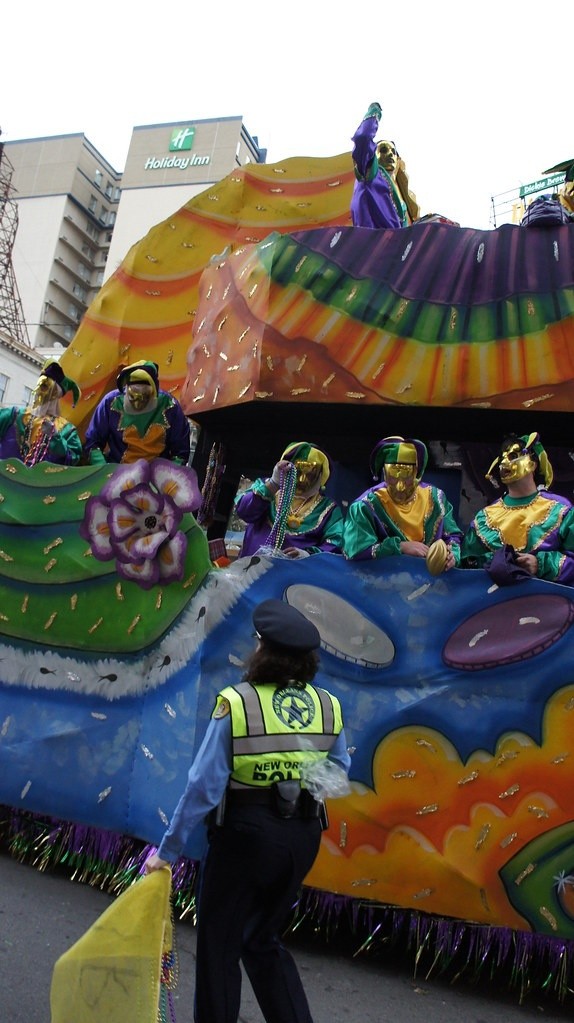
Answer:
[542,159,574,181]
[370,436,428,487]
[39,358,82,408]
[279,441,332,489]
[117,359,158,399]
[485,432,554,490]
[250,600,322,650]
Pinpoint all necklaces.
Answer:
[196,441,230,527]
[21,412,56,468]
[288,497,309,529]
[264,462,297,557]
[136,885,178,1023]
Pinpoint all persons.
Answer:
[235,442,344,559]
[520,158,574,227]
[460,432,574,587]
[344,436,464,571]
[0,357,82,468]
[231,478,254,533]
[351,101,418,230]
[84,360,190,466]
[146,599,351,1023]
